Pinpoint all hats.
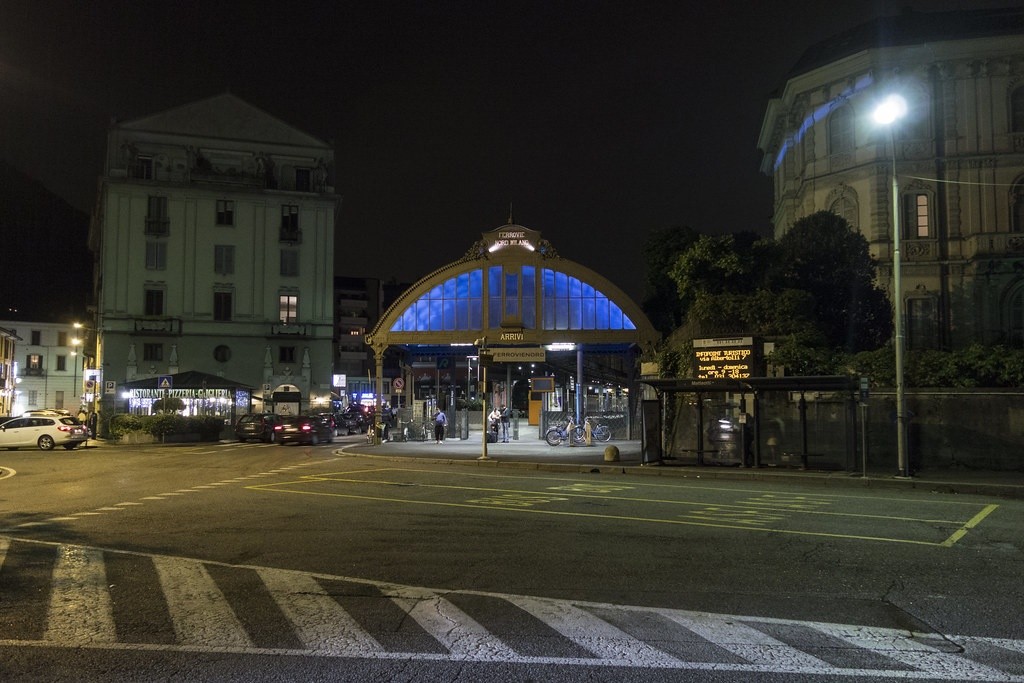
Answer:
[500,404,506,407]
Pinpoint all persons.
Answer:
[499,404,510,443]
[78,409,97,439]
[488,407,500,441]
[433,408,447,444]
[740,413,755,469]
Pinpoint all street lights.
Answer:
[71,338,90,369]
[73,317,98,414]
[868,92,910,478]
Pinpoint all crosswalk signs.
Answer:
[158,376,173,389]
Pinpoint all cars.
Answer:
[232,407,396,445]
[0,408,71,425]
[0,415,89,451]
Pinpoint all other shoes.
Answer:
[439,441,443,444]
[435,441,438,443]
[501,441,505,443]
[505,440,509,443]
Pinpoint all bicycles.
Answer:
[545,414,612,447]
[418,421,434,442]
[398,418,417,442]
